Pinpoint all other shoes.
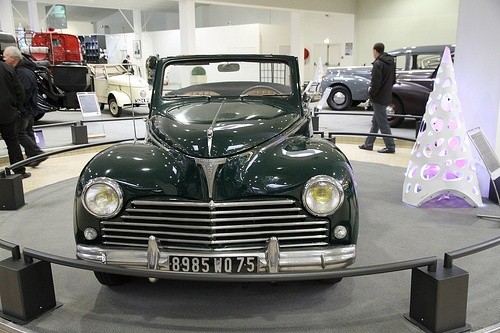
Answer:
[359,144,373,151]
[24,152,48,167]
[377,147,395,153]
[20,171,31,179]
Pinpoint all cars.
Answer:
[72,52,358,288]
[385,51,457,128]
[89,64,155,117]
[0,33,65,119]
[321,43,455,111]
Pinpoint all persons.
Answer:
[358,43,395,153]
[0,61,28,178]
[1,47,48,168]
[123,55,133,73]
[156,54,159,59]
